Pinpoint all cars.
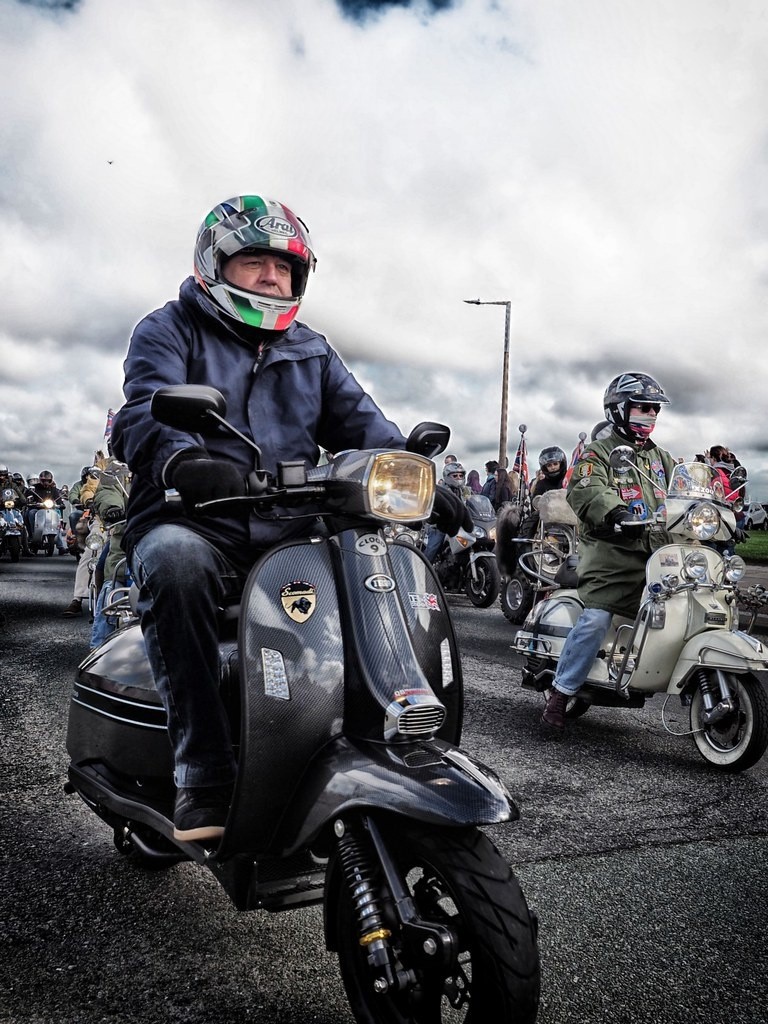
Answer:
[743,501,768,531]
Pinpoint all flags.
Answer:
[103,412,117,441]
[512,435,532,549]
[562,441,583,489]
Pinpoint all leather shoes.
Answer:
[540,687,569,735]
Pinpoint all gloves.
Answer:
[426,485,474,537]
[85,498,95,512]
[73,500,84,511]
[735,528,751,544]
[166,447,249,520]
[104,505,126,525]
[604,506,646,541]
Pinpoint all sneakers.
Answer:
[173,783,232,841]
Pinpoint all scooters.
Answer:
[0,481,142,633]
[62,382,544,1023]
[513,441,768,775]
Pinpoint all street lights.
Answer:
[461,298,511,471]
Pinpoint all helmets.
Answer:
[27,473,39,481]
[604,373,671,426]
[539,447,567,480]
[0,464,8,475]
[12,473,22,480]
[81,467,91,476]
[193,195,317,330]
[443,462,466,487]
[7,472,13,478]
[40,471,54,484]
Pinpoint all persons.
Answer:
[89,460,132,654]
[0,445,746,618]
[539,373,751,735]
[423,462,477,565]
[110,193,476,912]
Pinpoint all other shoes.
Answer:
[62,600,83,617]
[22,550,37,557]
[59,547,69,555]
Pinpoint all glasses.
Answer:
[452,475,463,478]
[630,403,660,414]
[41,479,52,484]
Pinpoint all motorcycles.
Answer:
[381,487,580,626]
